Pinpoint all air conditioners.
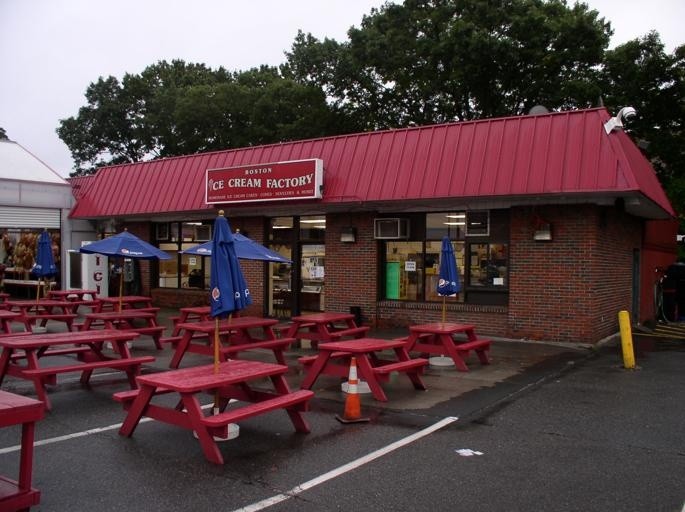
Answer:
[374,217,410,240]
[465,210,491,238]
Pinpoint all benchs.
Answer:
[330,326,371,341]
[296,351,353,364]
[393,333,434,343]
[456,339,491,350]
[372,357,429,383]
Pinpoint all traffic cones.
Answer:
[333,353,373,425]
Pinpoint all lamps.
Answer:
[533,206,552,241]
[340,212,357,243]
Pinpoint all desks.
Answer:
[402,322,491,371]
[281,311,359,351]
[300,337,427,403]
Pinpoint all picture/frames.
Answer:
[156,223,169,241]
[194,224,212,241]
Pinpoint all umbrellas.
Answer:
[209,209,254,416]
[31,227,57,325]
[76,227,173,331]
[178,229,293,341]
[436,236,461,358]
